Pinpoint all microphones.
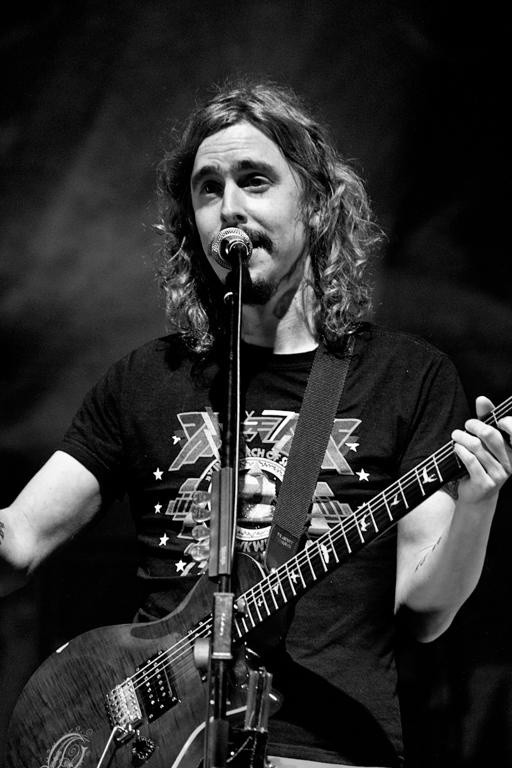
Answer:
[211,228,253,269]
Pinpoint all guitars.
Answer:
[6,397,510,762]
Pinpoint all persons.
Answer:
[0,75,512,767]
